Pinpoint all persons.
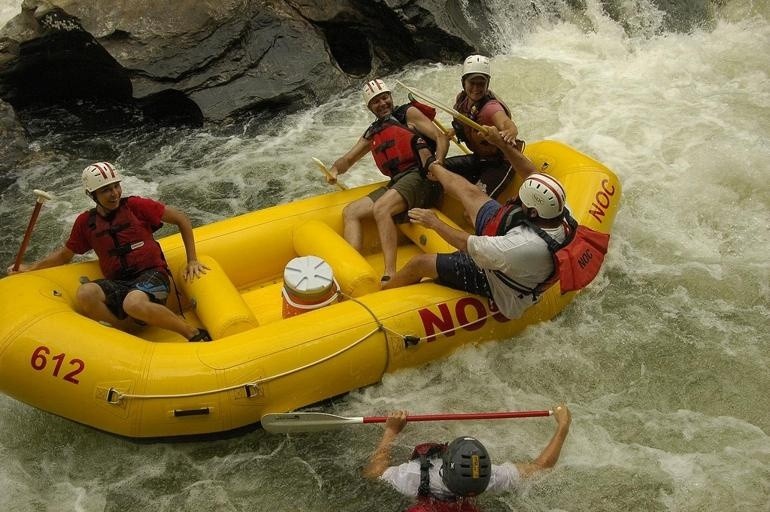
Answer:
[439,54,527,227]
[364,403,573,512]
[325,79,450,288]
[381,122,610,321]
[6,161,213,342]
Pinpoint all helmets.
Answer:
[81,161,121,194]
[461,53,490,79]
[518,172,566,219]
[362,79,392,105]
[443,435,492,496]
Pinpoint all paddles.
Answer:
[396,79,490,136]
[261,409,560,434]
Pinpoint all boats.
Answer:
[2,135,626,447]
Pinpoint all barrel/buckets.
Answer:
[281,255,341,320]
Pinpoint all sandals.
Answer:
[189,328,211,341]
[410,134,437,180]
